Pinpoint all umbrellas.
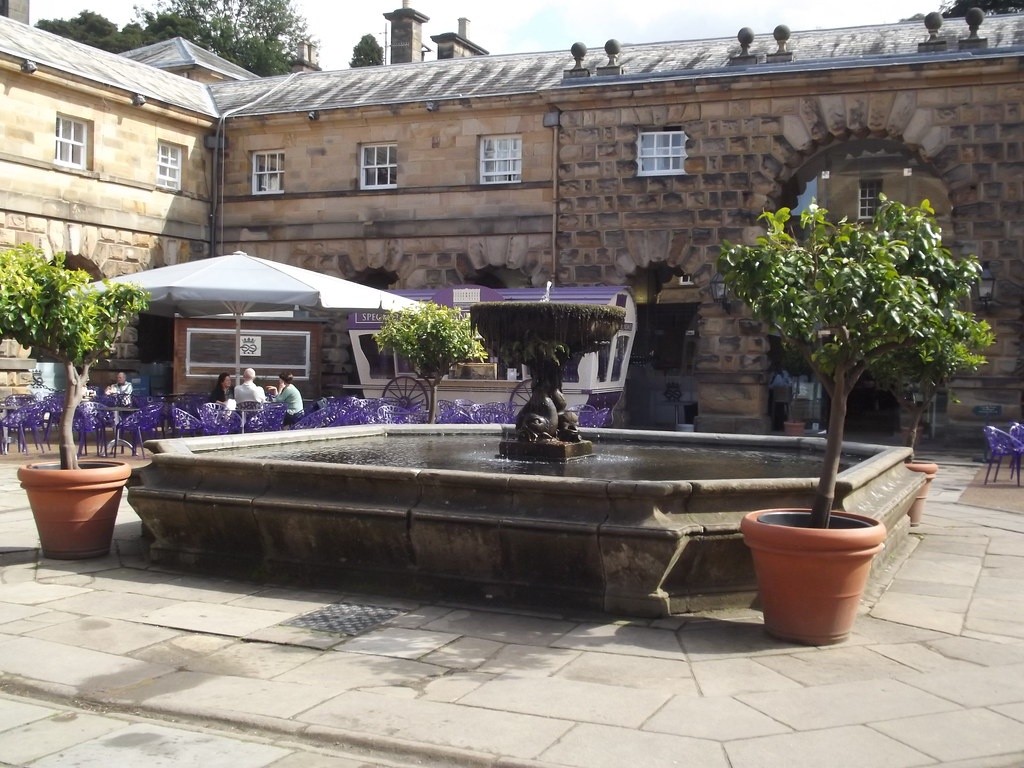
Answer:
[68,252,440,386]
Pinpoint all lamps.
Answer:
[132,93,147,107]
[22,59,38,74]
[309,111,318,120]
[543,106,561,127]
[426,102,439,111]
[974,260,994,312]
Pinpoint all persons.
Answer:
[234,368,265,426]
[76,371,94,408]
[768,369,790,430]
[266,373,305,426]
[210,372,231,419]
[105,371,132,407]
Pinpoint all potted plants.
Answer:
[781,337,812,435]
[872,308,998,526]
[0,243,148,561]
[716,191,981,647]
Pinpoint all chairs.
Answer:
[981,420,1024,486]
[0,385,610,459]
